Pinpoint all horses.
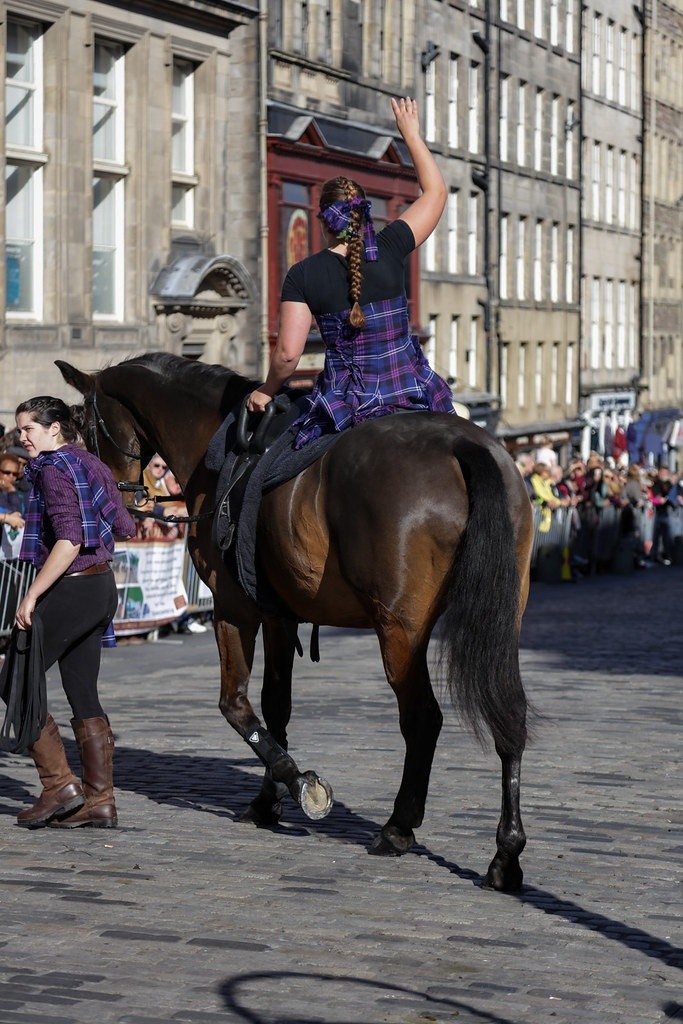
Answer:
[52,351,533,892]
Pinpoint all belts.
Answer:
[61,561,111,577]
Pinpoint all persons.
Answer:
[2,394,217,832]
[506,431,683,586]
[207,96,459,593]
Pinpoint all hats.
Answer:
[7,447,30,460]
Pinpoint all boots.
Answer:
[46,714,118,827]
[17,711,86,826]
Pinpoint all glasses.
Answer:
[0,469,19,476]
[154,464,167,469]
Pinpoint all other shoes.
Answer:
[173,621,213,634]
[115,636,144,646]
